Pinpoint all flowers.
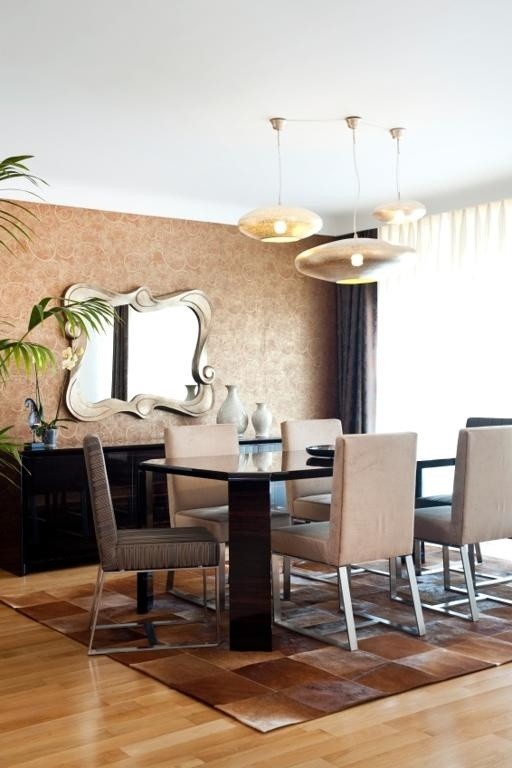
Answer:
[24,343,84,447]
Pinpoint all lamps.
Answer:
[372,128,428,227]
[238,119,323,243]
[294,115,418,285]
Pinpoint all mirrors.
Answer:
[63,282,216,422]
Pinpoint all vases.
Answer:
[216,384,248,437]
[252,402,272,438]
[42,429,56,448]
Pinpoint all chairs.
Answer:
[413,424,512,625]
[165,422,293,613]
[84,433,225,655]
[414,417,512,565]
[266,430,426,652]
[281,420,344,603]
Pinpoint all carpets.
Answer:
[0,544,512,732]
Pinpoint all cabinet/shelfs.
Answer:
[0,437,281,575]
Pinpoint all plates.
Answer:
[306,444,335,458]
